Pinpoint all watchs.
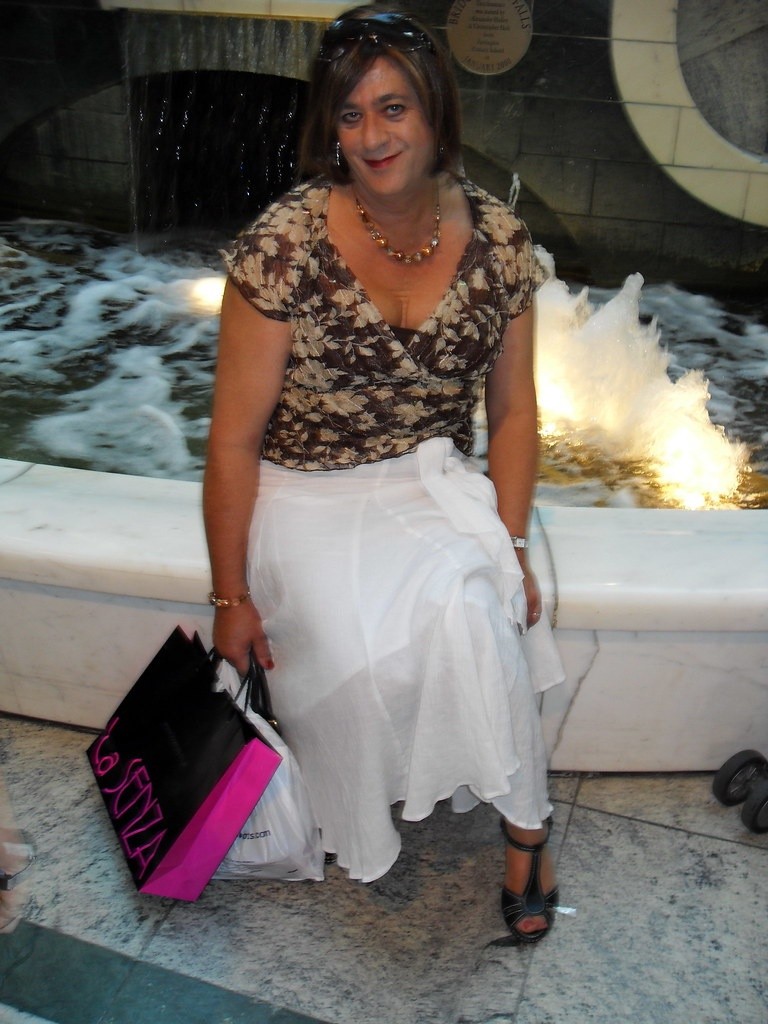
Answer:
[511,537,528,548]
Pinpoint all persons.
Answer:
[201,5,560,944]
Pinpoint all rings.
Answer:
[533,612,540,616]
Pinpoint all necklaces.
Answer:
[352,172,441,264]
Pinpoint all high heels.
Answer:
[499,813,559,943]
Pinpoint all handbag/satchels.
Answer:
[84,623,326,903]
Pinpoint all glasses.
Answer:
[319,13,436,63]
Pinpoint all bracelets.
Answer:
[208,591,251,607]
